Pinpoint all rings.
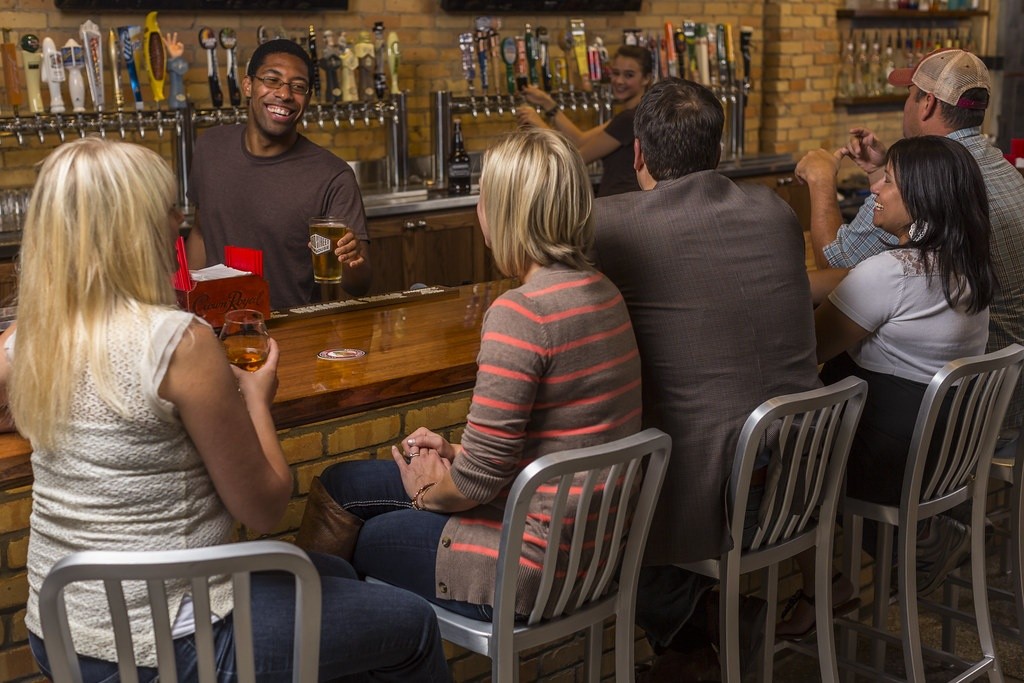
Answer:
[410,453,420,457]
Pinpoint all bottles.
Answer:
[448,119,472,197]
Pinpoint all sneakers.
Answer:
[948,505,995,546]
[889,513,973,599]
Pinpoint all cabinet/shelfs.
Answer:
[833,7,990,110]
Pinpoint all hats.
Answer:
[888,47,993,110]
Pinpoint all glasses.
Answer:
[248,74,313,95]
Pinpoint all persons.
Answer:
[795,48,1024,600]
[578,77,820,683]
[0,137,447,683]
[774,137,993,640]
[514,44,654,201]
[293,129,641,626]
[186,41,372,311]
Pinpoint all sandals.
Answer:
[774,571,863,640]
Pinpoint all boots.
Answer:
[294,475,365,561]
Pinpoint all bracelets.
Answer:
[412,482,437,512]
[544,104,561,117]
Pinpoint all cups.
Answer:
[308,214,348,284]
[219,308,270,373]
[0,184,31,217]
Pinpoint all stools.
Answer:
[870,412,1024,674]
[365,425,673,683]
[585,376,869,683]
[39,540,323,683]
[757,342,1024,683]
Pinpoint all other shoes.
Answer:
[708,590,769,683]
[635,640,723,683]
